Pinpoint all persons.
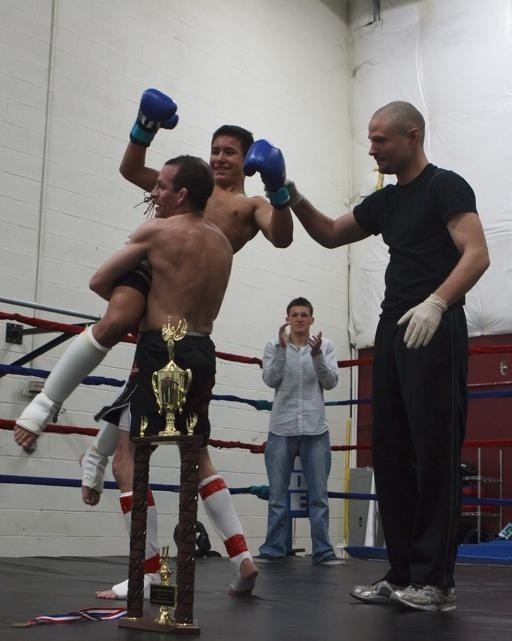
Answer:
[91,155,259,600]
[263,101,491,612]
[14,87,294,505]
[258,296,345,566]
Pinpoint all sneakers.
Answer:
[315,551,347,566]
[250,552,286,563]
[349,578,405,605]
[391,581,457,612]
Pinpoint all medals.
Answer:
[13,618,32,630]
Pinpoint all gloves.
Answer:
[129,88,179,147]
[243,139,291,208]
[264,178,304,209]
[397,294,449,350]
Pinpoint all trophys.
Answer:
[120,314,204,633]
